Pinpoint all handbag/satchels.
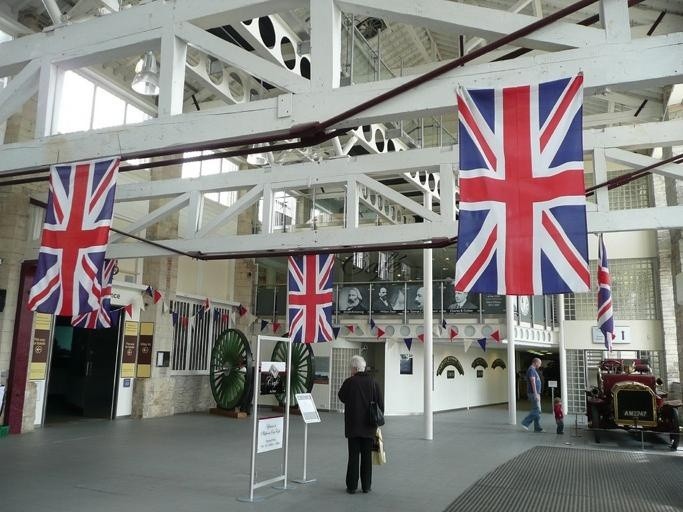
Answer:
[373,428,385,465]
[365,372,386,427]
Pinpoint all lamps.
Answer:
[246,79,276,166]
[130,50,159,97]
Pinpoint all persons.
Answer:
[335,353,384,494]
[447,290,477,309]
[413,286,424,310]
[519,358,546,433]
[343,286,368,311]
[553,397,564,434]
[371,287,394,311]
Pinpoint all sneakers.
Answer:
[520,423,529,431]
[534,429,546,433]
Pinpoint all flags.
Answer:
[454,71,591,296]
[70,258,111,329]
[287,253,335,343]
[25,155,121,316]
[597,233,615,352]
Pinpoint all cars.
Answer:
[585,356,683,451]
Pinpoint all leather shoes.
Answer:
[363,487,372,493]
[346,488,355,494]
